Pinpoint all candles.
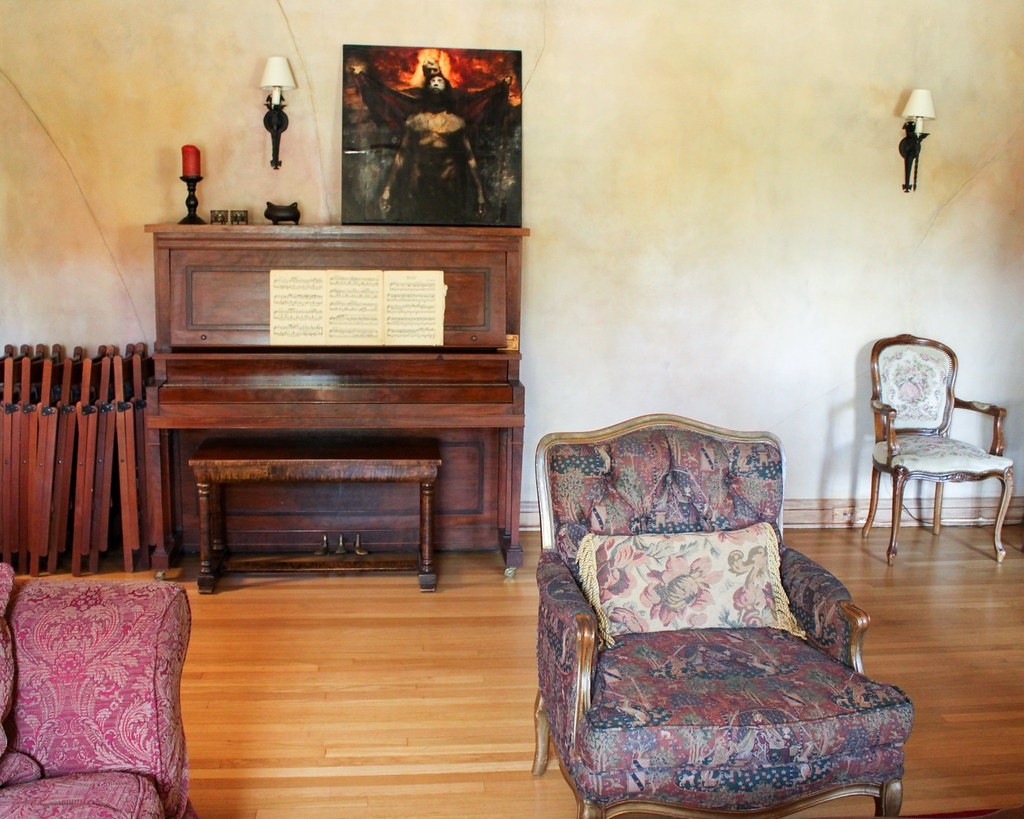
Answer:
[181,145,201,176]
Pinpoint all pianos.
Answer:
[139,217,529,582]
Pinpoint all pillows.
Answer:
[0,561,42,788]
[574,523,807,652]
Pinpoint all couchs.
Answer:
[0,578,193,819]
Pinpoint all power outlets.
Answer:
[834,507,858,523]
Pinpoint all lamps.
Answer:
[260,56,297,169]
[898,89,936,192]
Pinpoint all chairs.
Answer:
[531,413,914,819]
[0,340,175,577]
[862,333,1015,568]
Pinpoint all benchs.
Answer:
[188,437,443,596]
